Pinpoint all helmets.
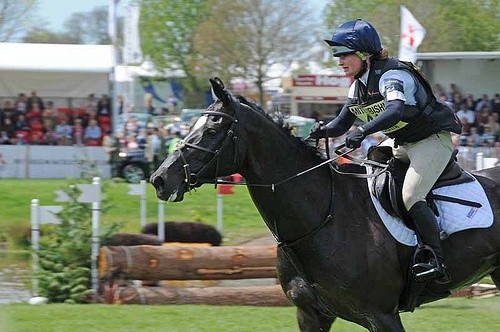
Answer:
[325,17,383,60]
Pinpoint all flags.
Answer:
[124,5,144,65]
[107,1,121,64]
[398,4,426,66]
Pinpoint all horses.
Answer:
[151,76,500,332]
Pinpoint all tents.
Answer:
[0,42,116,182]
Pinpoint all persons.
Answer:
[1,92,182,182]
[434,84,499,169]
[310,19,455,284]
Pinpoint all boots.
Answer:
[408,200,442,280]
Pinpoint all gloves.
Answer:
[345,126,366,149]
[308,123,331,139]
